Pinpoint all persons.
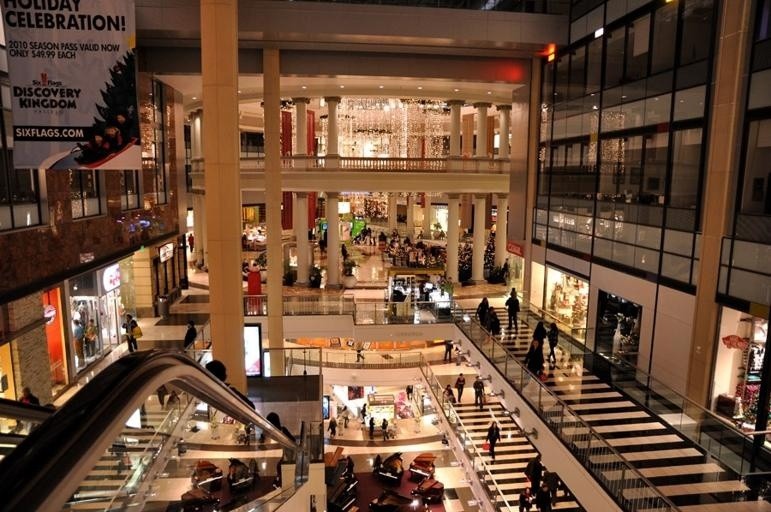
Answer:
[355,342,365,362]
[473,375,484,410]
[324,229,328,247]
[444,339,453,363]
[342,243,348,260]
[416,230,423,239]
[327,417,337,438]
[16,387,39,436]
[532,321,546,347]
[445,384,456,403]
[360,224,366,241]
[484,306,496,332]
[380,418,389,442]
[182,321,197,349]
[165,391,179,405]
[122,312,139,352]
[444,390,453,404]
[176,437,186,458]
[546,322,559,360]
[202,360,258,411]
[368,417,375,440]
[505,292,521,331]
[361,402,367,423]
[155,384,168,410]
[366,227,373,245]
[379,231,386,241]
[372,455,383,474]
[72,319,86,369]
[243,424,250,445]
[542,470,560,507]
[529,455,544,496]
[519,487,533,511]
[188,233,194,253]
[485,421,500,459]
[386,240,397,266]
[258,411,296,489]
[489,313,501,336]
[83,319,97,357]
[406,385,411,400]
[341,405,349,428]
[523,340,545,380]
[319,236,327,257]
[111,433,127,473]
[456,373,465,403]
[345,456,354,480]
[536,483,553,512]
[477,296,489,331]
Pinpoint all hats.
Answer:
[189,320,195,326]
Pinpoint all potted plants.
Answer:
[342,261,357,288]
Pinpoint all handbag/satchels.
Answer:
[131,326,143,339]
[455,383,458,388]
[483,443,490,450]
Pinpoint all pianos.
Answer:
[189,461,223,494]
[184,489,221,512]
[324,447,344,486]
[370,489,413,511]
[338,495,356,510]
[343,478,358,492]
[408,453,437,483]
[417,476,444,504]
[227,459,253,491]
[374,452,404,486]
